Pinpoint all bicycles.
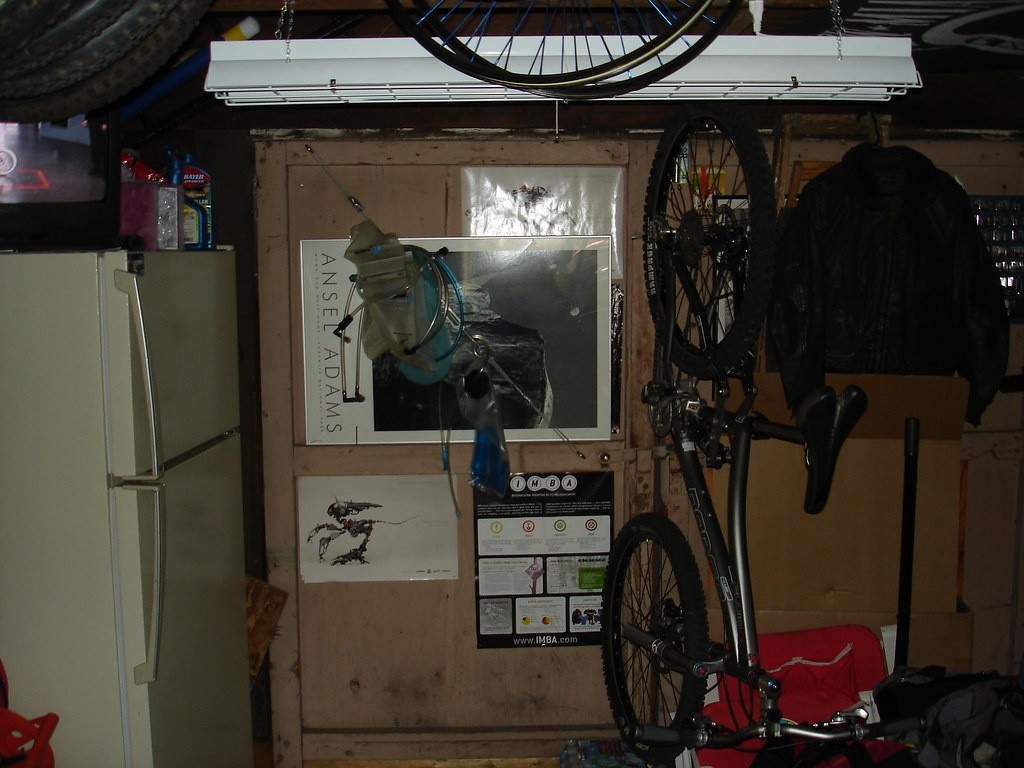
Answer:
[583,106,925,765]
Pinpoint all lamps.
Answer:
[202,0,922,108]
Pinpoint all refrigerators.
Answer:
[0,250,257,765]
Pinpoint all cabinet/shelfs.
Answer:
[254,128,1024,768]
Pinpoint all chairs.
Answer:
[691,624,924,768]
